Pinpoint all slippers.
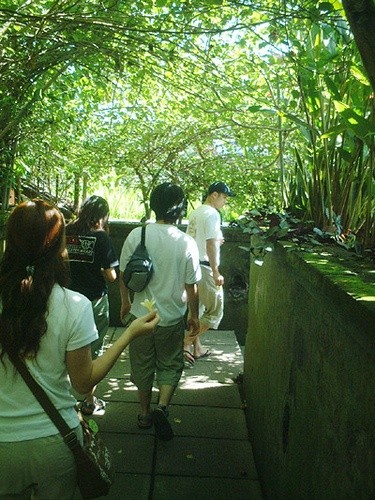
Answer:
[192,348,212,359]
[181,348,197,367]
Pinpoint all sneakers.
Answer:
[134,413,153,430]
[153,402,172,441]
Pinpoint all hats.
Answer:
[207,180,238,197]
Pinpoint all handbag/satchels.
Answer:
[71,417,115,499]
[122,225,155,294]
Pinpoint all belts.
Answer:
[200,261,210,266]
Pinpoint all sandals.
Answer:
[77,397,105,416]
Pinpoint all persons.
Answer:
[187,182,238,362]
[67,196,117,416]
[119,183,201,441]
[0,198,162,500]
